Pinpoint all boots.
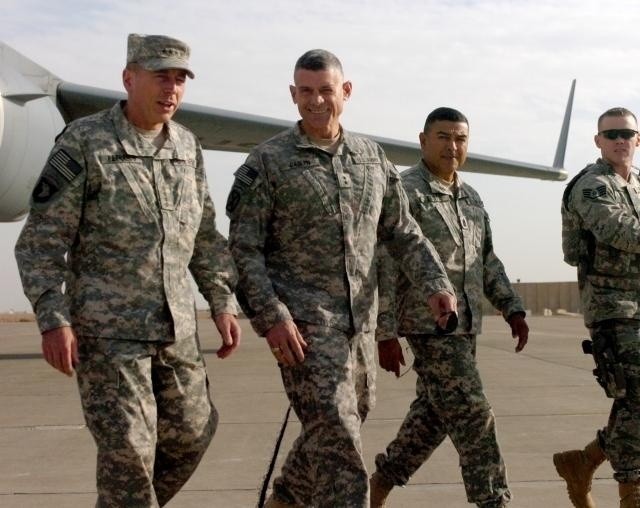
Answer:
[619,482,640,508]
[264,494,288,508]
[553,438,607,508]
[369,471,394,508]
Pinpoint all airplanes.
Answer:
[0,41,577,222]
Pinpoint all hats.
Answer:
[127,33,195,79]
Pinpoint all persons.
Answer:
[367,107,530,508]
[224,49,458,508]
[553,107,639,507]
[13,31,240,508]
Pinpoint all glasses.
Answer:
[598,129,638,139]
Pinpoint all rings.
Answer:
[271,347,280,354]
